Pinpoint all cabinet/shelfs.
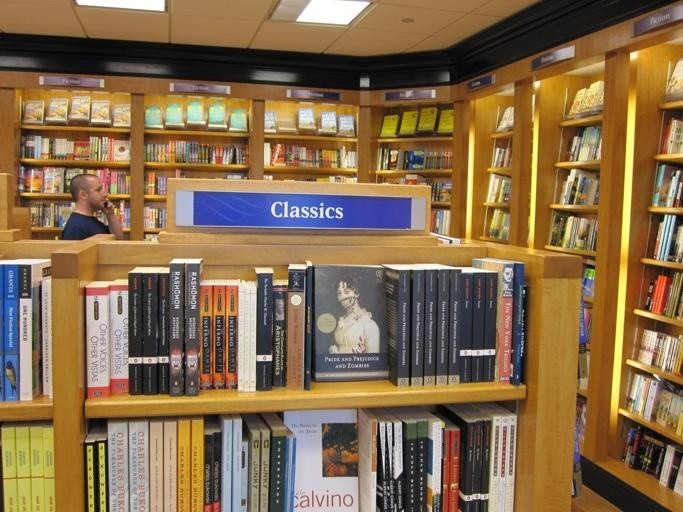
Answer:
[264,99,358,182]
[77,241,534,511]
[466,78,537,241]
[531,54,602,456]
[374,103,455,240]
[20,90,131,240]
[1,243,70,512]
[141,94,250,225]
[604,29,681,496]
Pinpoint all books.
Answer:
[567,126,601,161]
[653,214,682,261]
[187,102,203,122]
[264,111,276,129]
[497,106,514,131]
[321,111,336,130]
[114,103,131,123]
[1,422,57,511]
[338,114,355,137]
[298,109,315,130]
[70,168,100,178]
[89,100,111,119]
[2,258,51,403]
[433,182,452,202]
[438,110,454,133]
[264,142,356,168]
[18,166,70,193]
[144,138,249,166]
[380,173,433,185]
[626,372,683,438]
[632,327,683,377]
[662,118,683,154]
[145,105,160,125]
[493,148,511,168]
[622,426,683,496]
[69,96,90,116]
[84,260,312,397]
[417,107,437,130]
[665,57,683,101]
[398,111,418,135]
[314,264,388,380]
[228,109,247,129]
[381,115,400,138]
[93,210,108,227]
[384,257,525,386]
[175,168,247,179]
[145,234,158,242]
[46,98,68,117]
[20,135,130,162]
[653,164,683,207]
[558,169,600,205]
[24,202,75,227]
[567,80,604,117]
[115,208,130,229]
[101,169,132,194]
[377,144,452,169]
[489,208,511,241]
[143,206,166,228]
[550,213,597,251]
[22,99,45,120]
[165,102,183,123]
[263,175,357,184]
[431,208,451,236]
[208,103,225,124]
[646,273,683,321]
[144,171,166,195]
[488,173,511,203]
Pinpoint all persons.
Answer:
[60,173,123,240]
[329,273,380,354]
